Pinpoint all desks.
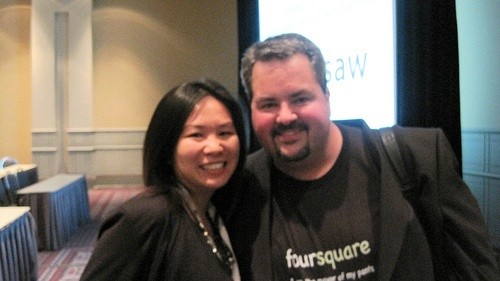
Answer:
[16,174,90,251]
[0,164,39,207]
[0,205,38,281]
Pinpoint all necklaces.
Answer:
[179,184,235,265]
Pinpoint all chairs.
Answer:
[4,172,21,205]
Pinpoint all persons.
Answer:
[225,33,500,281]
[79,77,246,281]
